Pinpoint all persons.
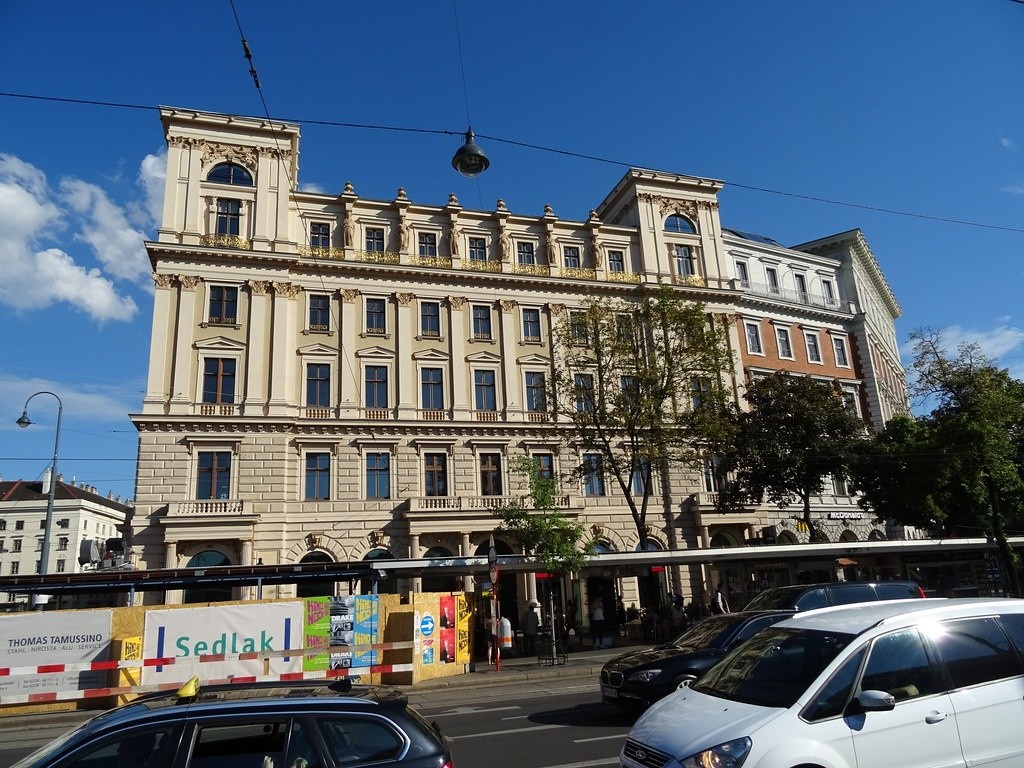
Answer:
[667,592,683,610]
[522,605,539,656]
[715,583,730,615]
[590,596,608,649]
[564,599,583,647]
[485,608,500,665]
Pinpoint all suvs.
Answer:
[6,674,457,768]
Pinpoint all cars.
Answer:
[617,595,1024,766]
[599,606,813,716]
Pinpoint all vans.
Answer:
[740,580,925,615]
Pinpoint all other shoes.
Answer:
[593,645,599,650]
[600,644,609,649]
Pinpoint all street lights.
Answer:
[15,390,64,576]
